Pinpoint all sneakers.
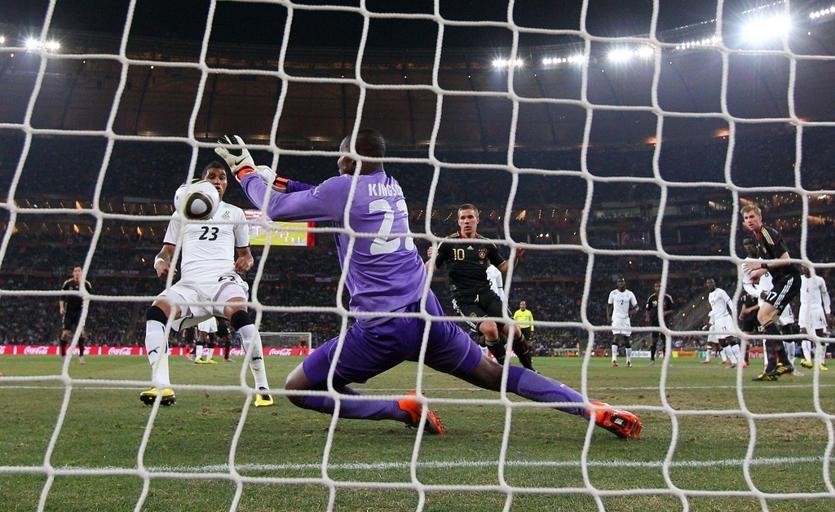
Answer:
[609,360,673,368]
[399,390,446,436]
[583,399,643,440]
[800,360,814,369]
[768,362,794,377]
[78,355,86,365]
[254,389,274,407]
[751,371,778,382]
[140,387,175,406]
[819,363,829,372]
[194,357,237,364]
[703,356,749,367]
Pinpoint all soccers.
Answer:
[172,178,221,218]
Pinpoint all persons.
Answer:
[58,266,90,365]
[645,280,675,364]
[424,204,536,369]
[703,261,834,370]
[452,253,507,350]
[0,191,834,356]
[737,197,795,381]
[513,300,534,362]
[183,313,233,363]
[606,275,640,367]
[214,131,646,439]
[139,160,273,409]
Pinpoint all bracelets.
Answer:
[760,262,767,267]
[153,257,164,269]
